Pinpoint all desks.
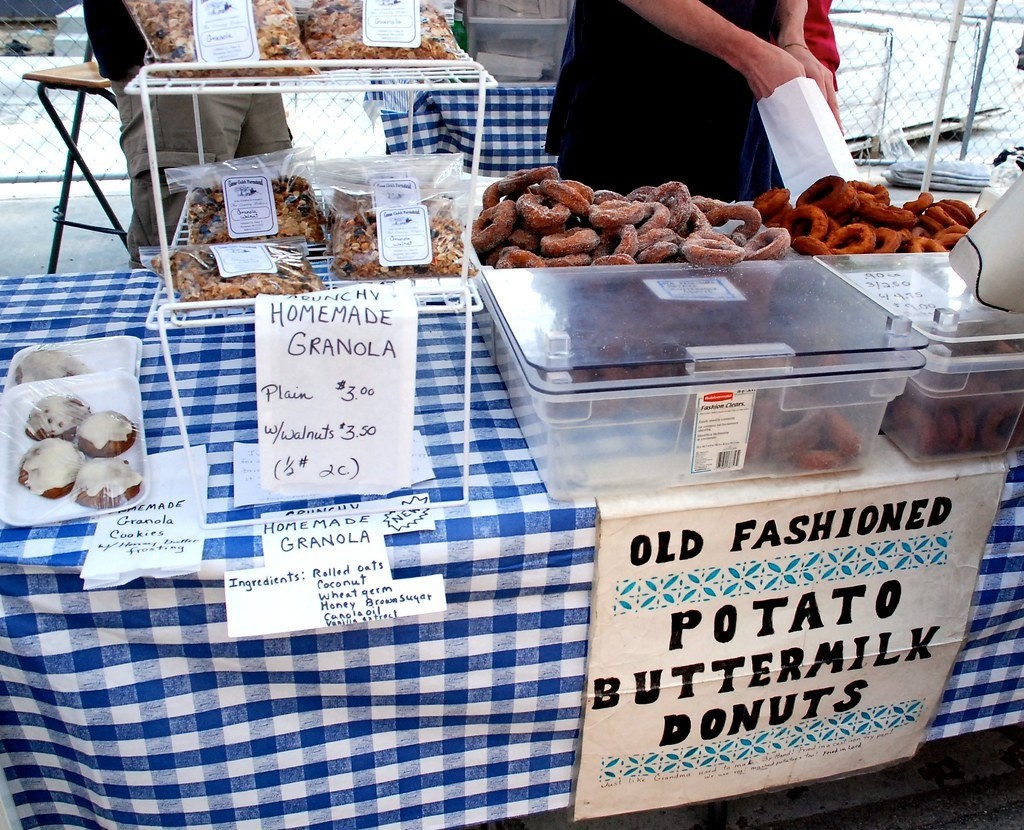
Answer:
[1,271,1023,830]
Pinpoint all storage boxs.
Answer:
[815,252,1024,464]
[470,15,568,81]
[474,271,930,500]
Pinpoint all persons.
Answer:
[83,0,295,270]
[544,0,840,234]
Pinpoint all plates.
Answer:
[5,336,143,383]
[0,370,149,526]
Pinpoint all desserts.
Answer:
[20,396,141,509]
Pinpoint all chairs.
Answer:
[24,40,131,274]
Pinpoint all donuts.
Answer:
[472,167,1020,467]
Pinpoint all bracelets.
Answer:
[782,42,808,51]
